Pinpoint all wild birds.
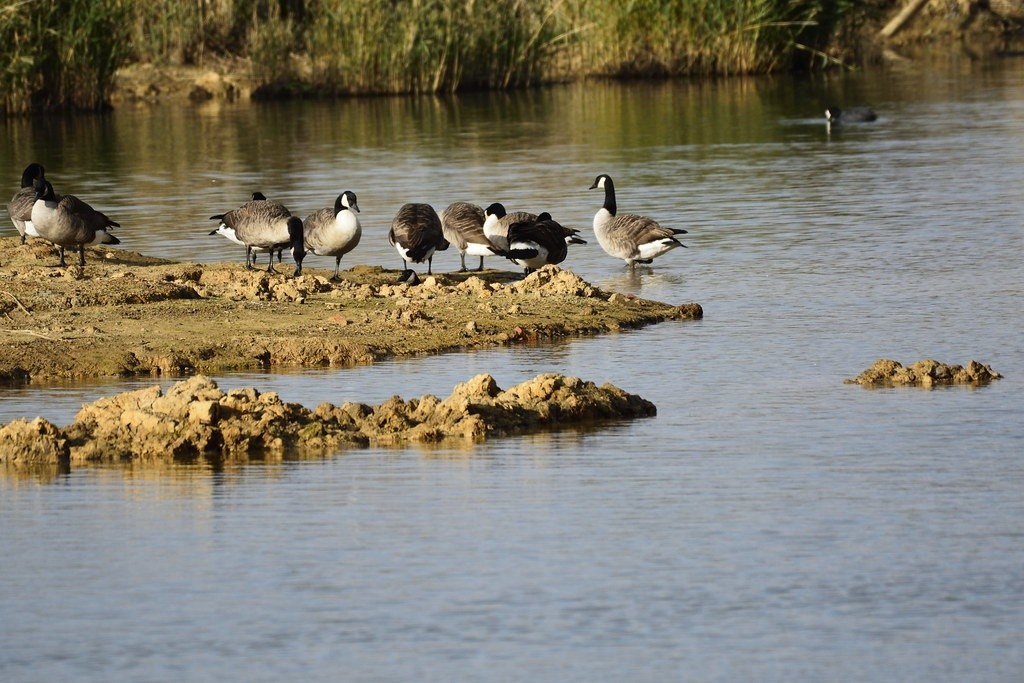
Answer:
[388,202,450,275]
[588,173,689,272]
[207,190,362,282]
[824,104,879,123]
[476,202,587,281]
[9,162,122,271]
[434,201,519,273]
